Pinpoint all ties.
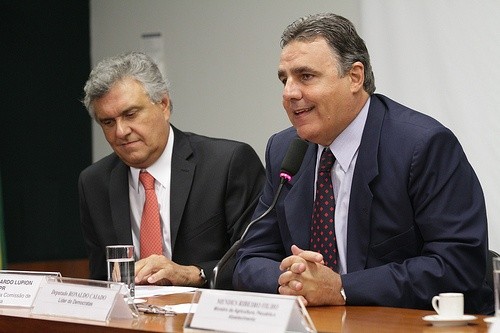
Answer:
[140,172,161,259]
[310,148,339,273]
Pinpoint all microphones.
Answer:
[209,138,310,288]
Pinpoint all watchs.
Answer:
[193,265,206,286]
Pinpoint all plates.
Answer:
[422,314,477,327]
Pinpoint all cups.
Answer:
[432,293,464,318]
[106,244,135,301]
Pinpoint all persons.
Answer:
[78,51,265,285]
[231,13,496,316]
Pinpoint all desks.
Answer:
[304,299,491,333]
